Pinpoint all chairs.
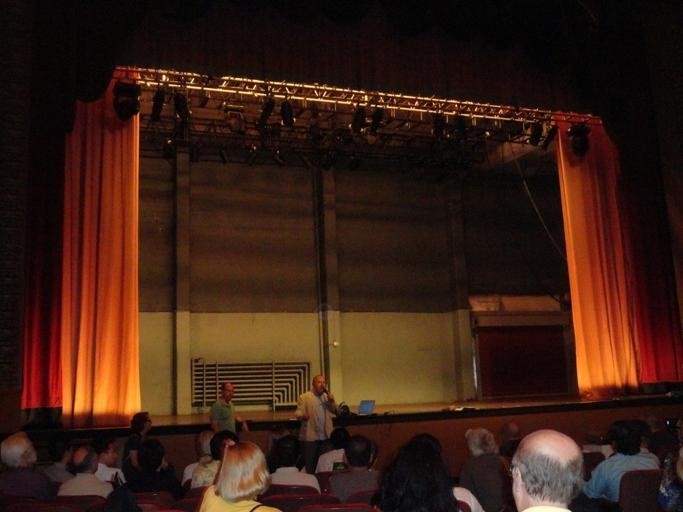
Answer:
[258,471,381,512]
[618,470,662,512]
[131,479,207,512]
[0,494,106,512]
[582,453,605,480]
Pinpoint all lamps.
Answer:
[371,109,384,131]
[434,113,444,134]
[352,108,366,132]
[543,128,557,146]
[281,102,293,127]
[173,93,189,120]
[529,124,542,146]
[150,89,165,121]
[261,99,275,121]
[455,116,467,138]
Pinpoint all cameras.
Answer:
[664,417,677,430]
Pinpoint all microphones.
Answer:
[322,387,329,395]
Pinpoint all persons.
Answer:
[294,374,337,473]
[0,411,682,512]
[208,380,249,437]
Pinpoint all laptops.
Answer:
[357,400,375,416]
